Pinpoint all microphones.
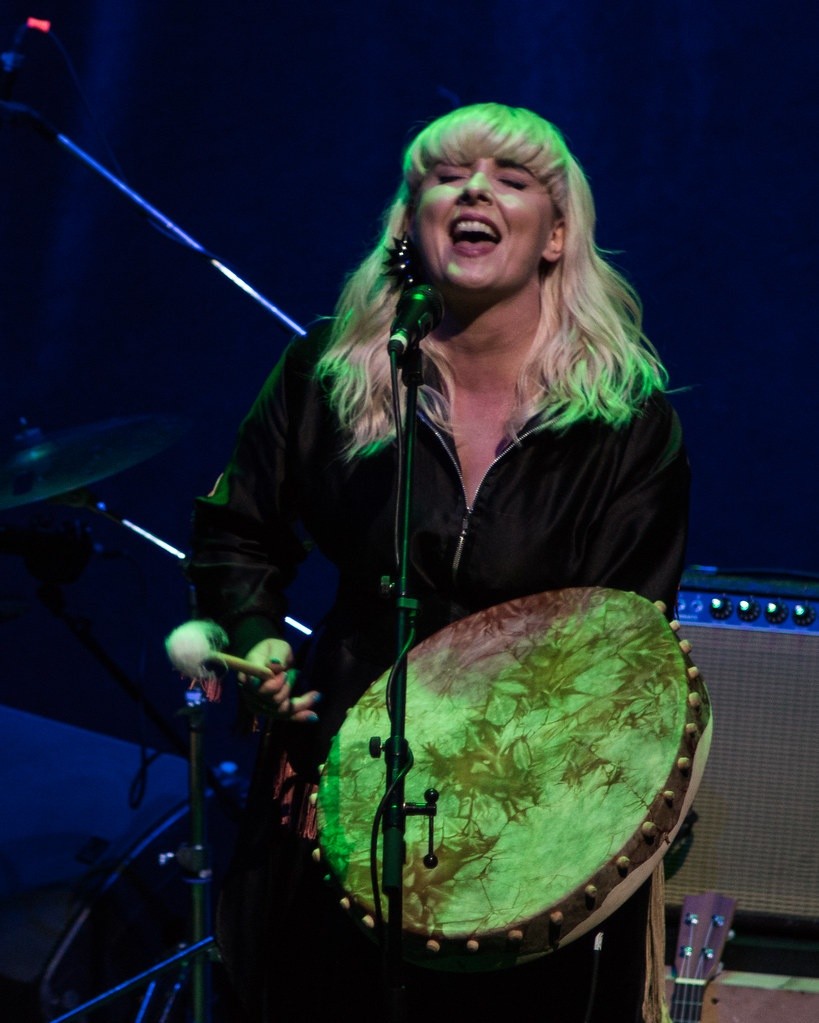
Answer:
[386,283,445,354]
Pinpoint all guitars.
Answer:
[670,892,738,1023]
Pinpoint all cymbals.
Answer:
[0,414,191,511]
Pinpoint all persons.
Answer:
[187,101,692,1023]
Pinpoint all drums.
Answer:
[310,583,716,974]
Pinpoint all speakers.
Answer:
[0,706,254,1023]
[652,622,818,979]
